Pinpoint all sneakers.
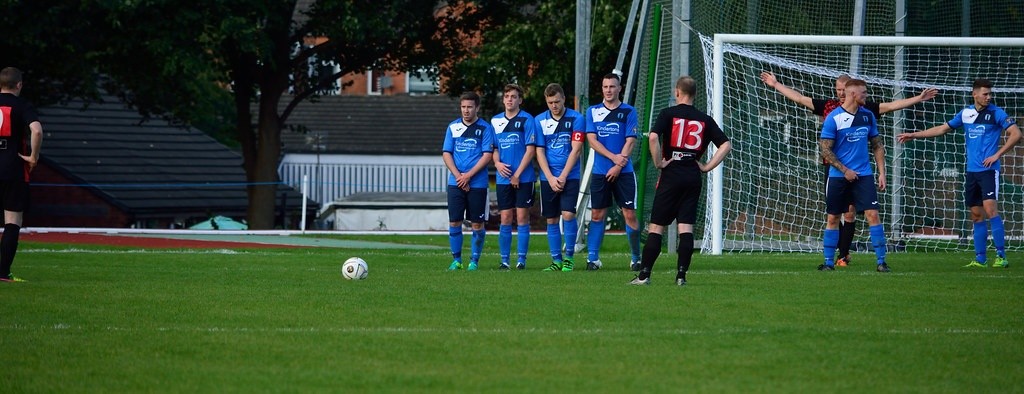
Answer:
[628,274,649,285]
[586,259,602,270]
[993,257,1009,268]
[562,257,574,271]
[676,279,686,286]
[818,264,835,271]
[467,260,478,270]
[542,262,562,271]
[878,263,891,272]
[836,256,847,267]
[449,260,463,270]
[630,260,642,271]
[845,255,851,264]
[499,262,511,270]
[516,262,525,268]
[0,273,28,283]
[962,259,988,267]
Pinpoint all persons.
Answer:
[535,83,586,272]
[760,71,939,267]
[584,73,641,271]
[819,79,892,272]
[630,76,731,286]
[0,67,44,282]
[490,84,537,269]
[442,92,494,270]
[896,78,1022,268]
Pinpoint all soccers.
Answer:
[341,256,368,281]
